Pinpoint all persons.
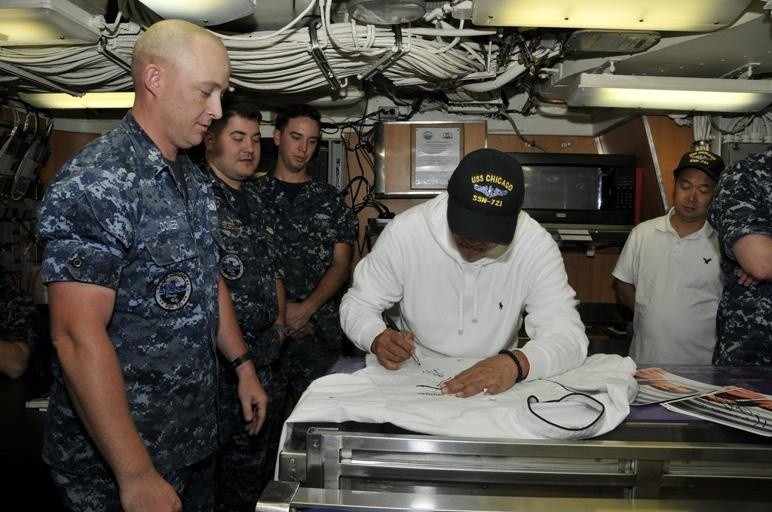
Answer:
[706,145,772,367]
[611,151,727,365]
[338,148,590,397]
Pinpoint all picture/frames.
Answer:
[409,124,465,191]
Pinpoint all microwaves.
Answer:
[492,152,639,231]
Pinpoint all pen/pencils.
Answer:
[381,311,421,366]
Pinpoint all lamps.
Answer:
[472,0,748,33]
[139,0,256,27]
[18,91,139,109]
[565,72,771,112]
[2,1,106,48]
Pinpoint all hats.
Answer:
[672,150,728,185]
[444,146,526,248]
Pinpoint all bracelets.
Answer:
[498,349,523,383]
